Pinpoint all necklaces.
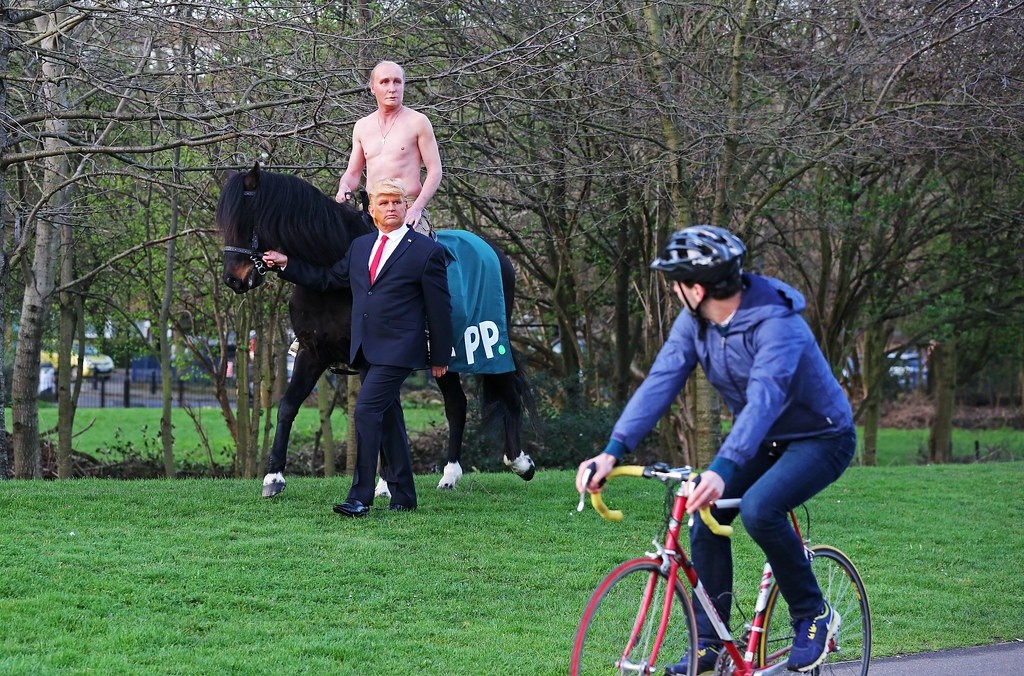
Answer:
[378,114,398,144]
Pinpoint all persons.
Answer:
[334,61,442,240]
[262,178,451,517]
[575,226,857,676]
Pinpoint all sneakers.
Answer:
[664,641,734,676]
[787,600,841,672]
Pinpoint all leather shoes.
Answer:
[334,499,370,516]
[375,501,415,514]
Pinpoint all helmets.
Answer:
[651,225,747,288]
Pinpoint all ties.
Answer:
[369,235,389,287]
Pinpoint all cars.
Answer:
[887,352,928,384]
[130,339,294,398]
[41,337,113,380]
[842,354,863,386]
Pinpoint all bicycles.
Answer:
[569,457,872,676]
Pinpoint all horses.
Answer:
[209,160,537,500]
